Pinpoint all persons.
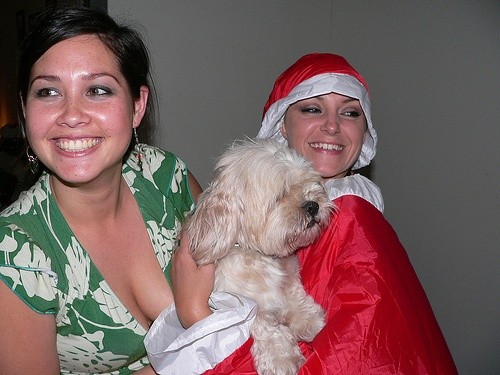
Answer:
[0,0,207,374]
[140,52,459,375]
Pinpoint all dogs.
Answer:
[175,133,340,375]
